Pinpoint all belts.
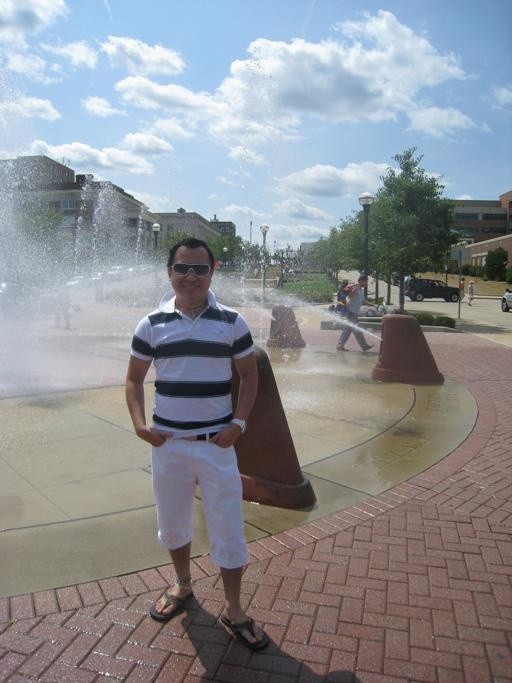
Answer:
[183,432,218,441]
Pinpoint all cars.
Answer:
[501,288,512,312]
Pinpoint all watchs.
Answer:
[230,419,247,434]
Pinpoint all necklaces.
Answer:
[177,302,206,319]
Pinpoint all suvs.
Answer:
[404,278,459,302]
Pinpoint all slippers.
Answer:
[150,582,193,622]
[220,611,269,650]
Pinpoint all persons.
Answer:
[467,281,474,306]
[329,279,357,315]
[460,277,466,303]
[336,274,374,351]
[125,238,269,651]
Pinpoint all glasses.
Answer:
[170,263,211,278]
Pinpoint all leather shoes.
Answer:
[363,345,374,351]
[336,347,350,351]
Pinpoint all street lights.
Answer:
[223,247,228,269]
[152,223,160,278]
[259,223,269,307]
[358,192,375,300]
